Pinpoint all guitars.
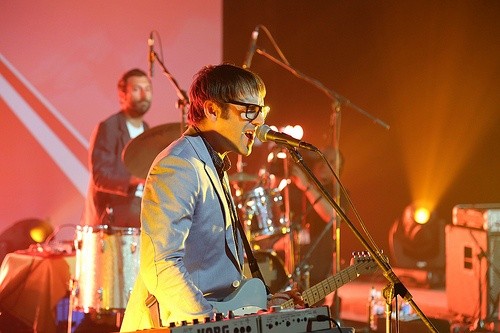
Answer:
[207,248,390,318]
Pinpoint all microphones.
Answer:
[148,32,153,77]
[255,125,318,151]
[245,27,258,68]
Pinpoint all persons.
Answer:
[84,68,153,228]
[119,62,304,333]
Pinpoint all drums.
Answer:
[70,224,142,314]
[243,250,291,295]
[233,188,274,241]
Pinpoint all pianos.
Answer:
[122,304,331,333]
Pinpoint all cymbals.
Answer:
[123,122,190,178]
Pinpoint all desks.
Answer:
[0,242,76,333]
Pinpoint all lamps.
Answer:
[387,201,447,268]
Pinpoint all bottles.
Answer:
[402,300,411,315]
[368,287,384,329]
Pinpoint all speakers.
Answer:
[445,224,500,323]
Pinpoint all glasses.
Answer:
[218,96,270,120]
